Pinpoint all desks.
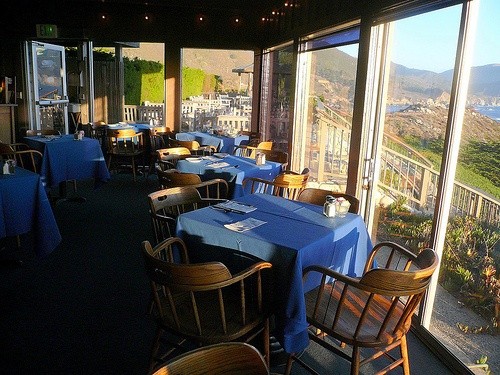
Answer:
[174,131,250,156]
[176,156,282,200]
[22,134,110,198]
[148,186,229,262]
[176,192,378,367]
[0,163,62,264]
[99,124,150,142]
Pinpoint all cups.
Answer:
[336,205,350,218]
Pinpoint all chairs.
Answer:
[148,341,270,375]
[0,119,311,202]
[294,188,360,214]
[141,236,275,371]
[285,240,438,375]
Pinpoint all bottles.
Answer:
[255,153,266,165]
[209,127,214,135]
[74,131,82,141]
[322,195,336,219]
